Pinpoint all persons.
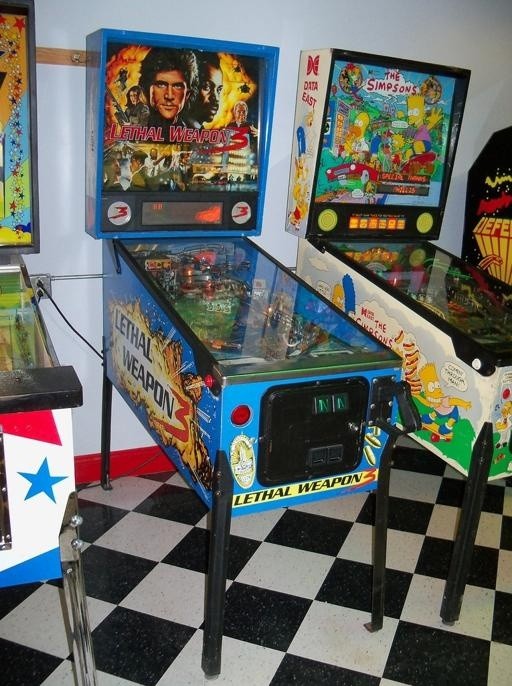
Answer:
[112,46,259,145]
[103,145,190,192]
